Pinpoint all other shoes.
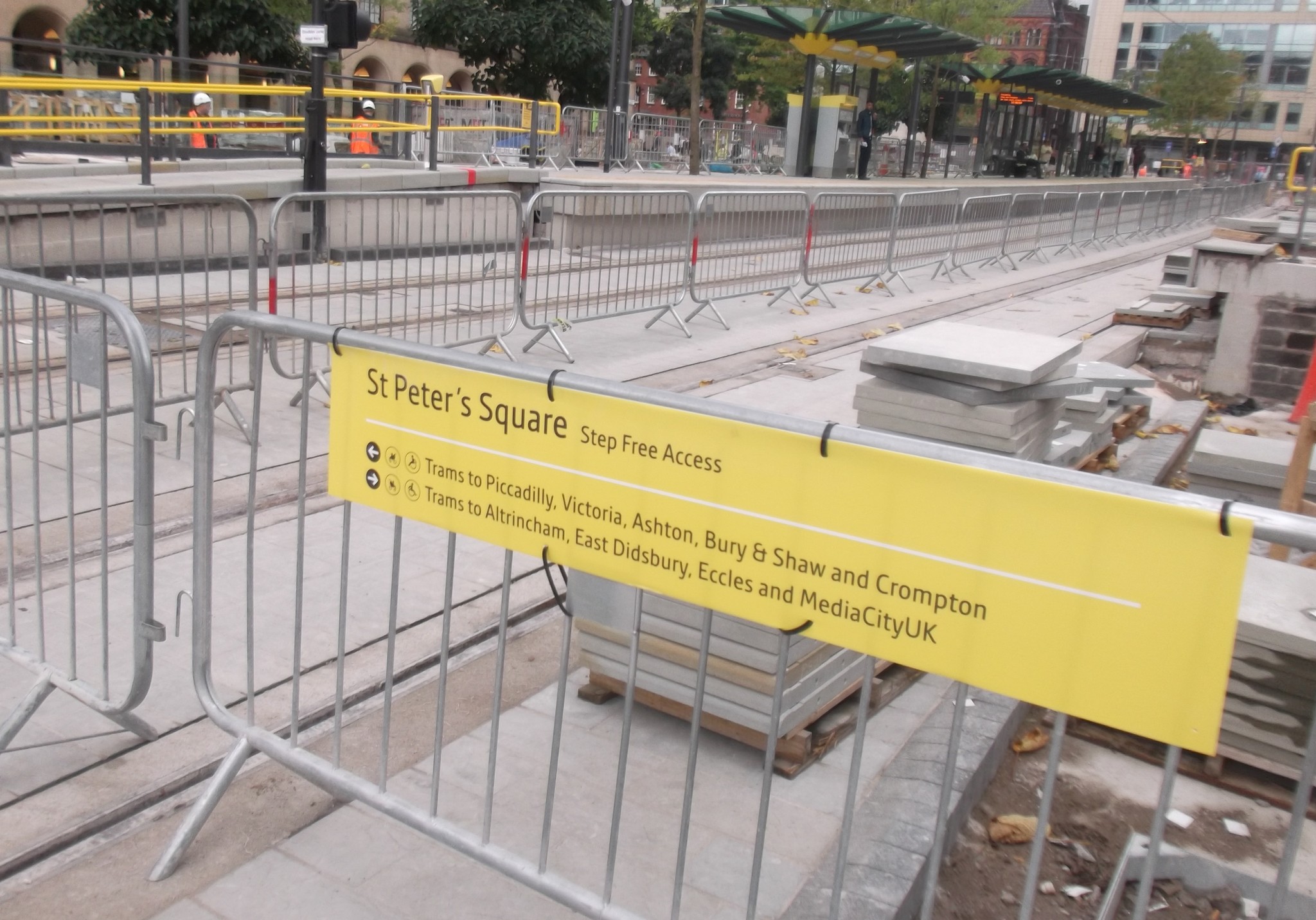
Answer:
[859,176,870,180]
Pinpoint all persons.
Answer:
[1014,143,1029,175]
[1040,139,1053,174]
[1139,166,1147,176]
[1133,141,1145,178]
[348,100,384,154]
[187,93,220,149]
[1191,152,1197,159]
[1182,162,1193,179]
[1093,139,1108,162]
[666,142,676,154]
[730,138,744,164]
[856,101,873,180]
[1112,146,1128,178]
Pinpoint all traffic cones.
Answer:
[1286,345,1316,427]
[877,144,889,176]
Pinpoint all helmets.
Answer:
[194,93,211,106]
[363,100,376,110]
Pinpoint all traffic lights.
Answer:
[318,0,371,49]
